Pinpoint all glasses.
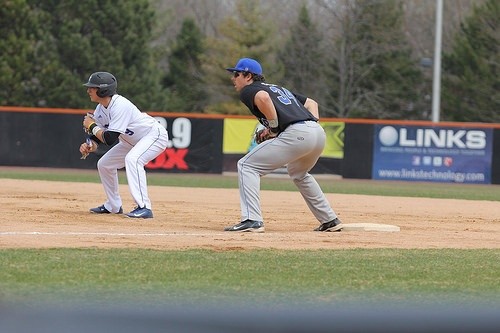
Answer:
[232,72,247,78]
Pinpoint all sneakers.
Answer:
[89,205,122,214]
[223,220,265,234]
[314,218,343,232]
[124,207,153,218]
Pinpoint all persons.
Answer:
[78,72,169,220]
[224,58,344,234]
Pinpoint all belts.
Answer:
[292,120,306,124]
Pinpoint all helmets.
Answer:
[83,72,118,98]
[226,58,263,75]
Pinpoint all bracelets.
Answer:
[88,122,101,136]
